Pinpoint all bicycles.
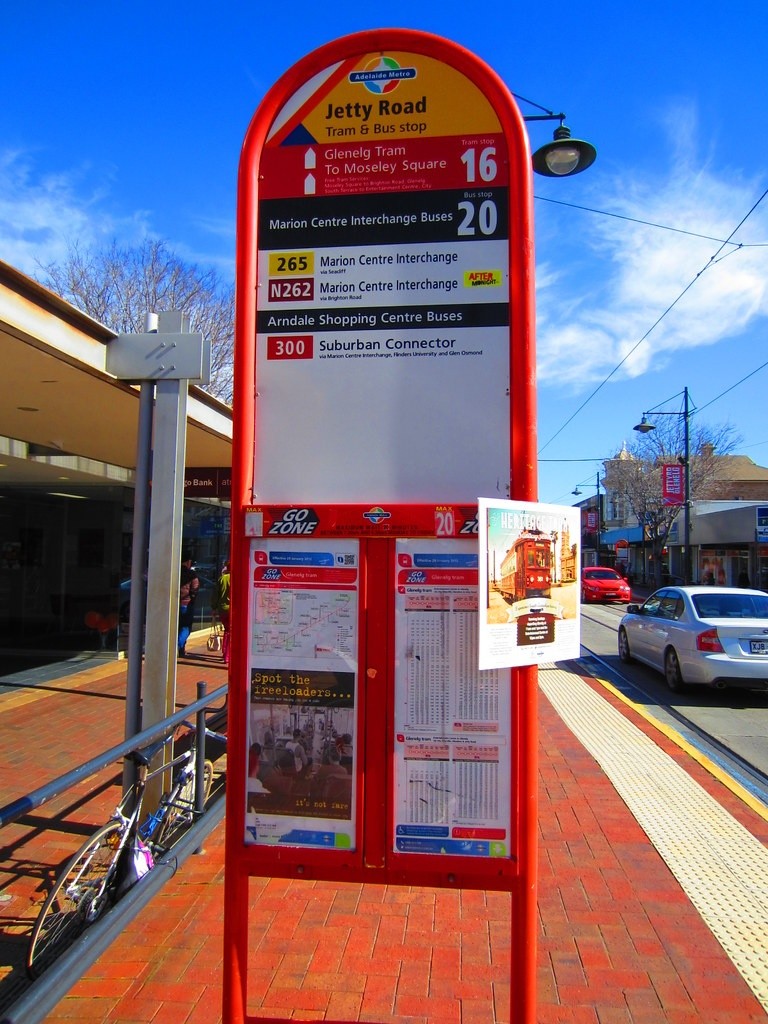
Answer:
[24,718,228,981]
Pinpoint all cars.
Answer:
[617,584,768,695]
[581,566,631,605]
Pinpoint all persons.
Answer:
[177,549,200,657]
[736,567,751,587]
[616,560,624,574]
[716,566,726,585]
[662,564,670,586]
[247,714,352,802]
[702,566,714,586]
[208,561,229,667]
[625,561,634,588]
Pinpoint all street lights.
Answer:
[633,387,697,585]
[573,472,601,567]
